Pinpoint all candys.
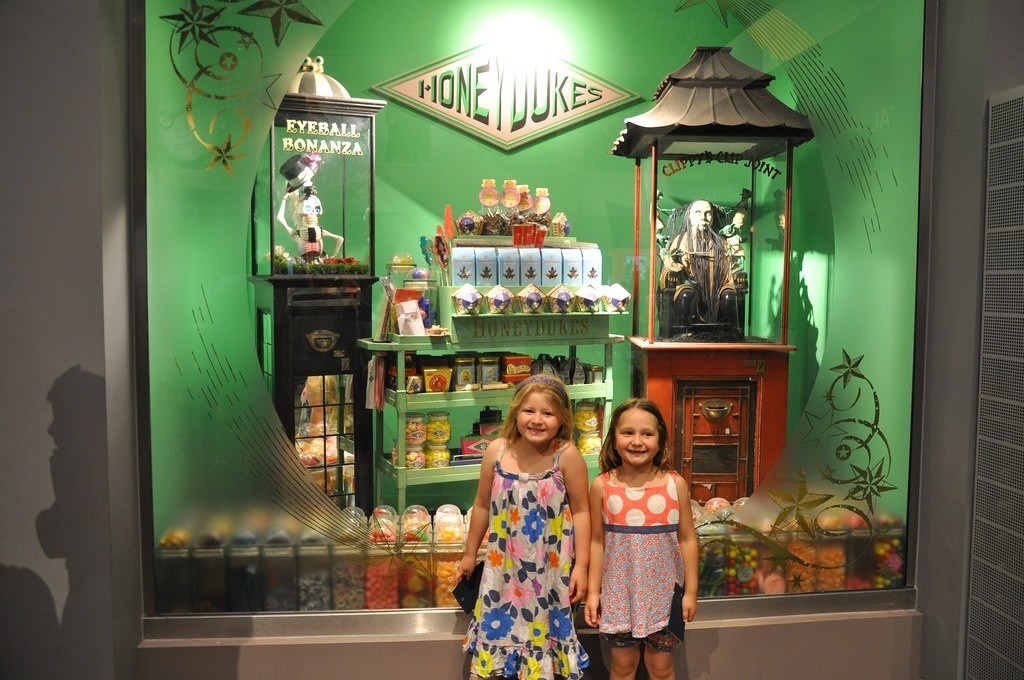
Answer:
[392,417,451,469]
[692,498,904,598]
[297,507,487,614]
[575,410,601,454]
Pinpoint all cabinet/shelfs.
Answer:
[613,42,815,509]
[249,58,387,511]
[357,334,629,518]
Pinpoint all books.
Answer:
[667,581,685,642]
[451,560,484,615]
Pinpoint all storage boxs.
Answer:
[460,437,497,460]
[450,248,603,285]
[424,354,532,392]
[473,423,503,437]
[480,410,502,423]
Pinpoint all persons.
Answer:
[585,398,699,680]
[451,372,591,680]
[659,199,741,332]
